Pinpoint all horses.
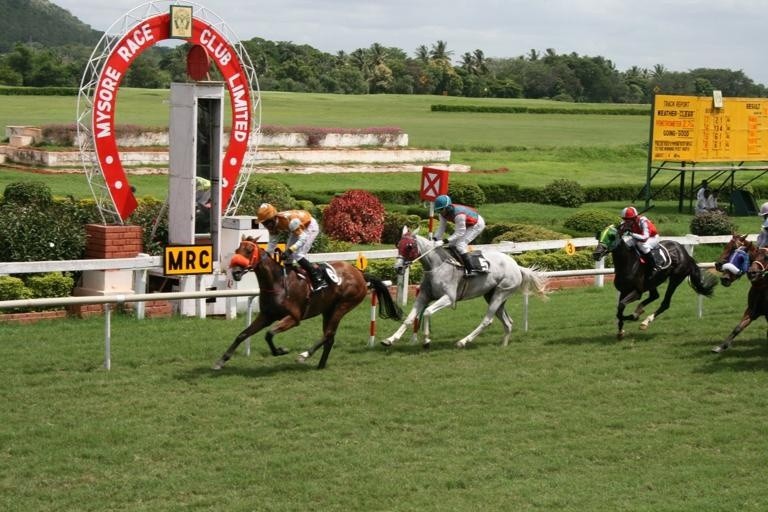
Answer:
[380,224,556,349]
[711,230,768,354]
[210,234,408,372]
[591,224,721,341]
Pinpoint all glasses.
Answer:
[440,208,445,211]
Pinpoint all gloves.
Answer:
[624,231,633,236]
[434,239,449,247]
[280,249,293,260]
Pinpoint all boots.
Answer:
[645,251,661,271]
[460,252,482,277]
[313,271,329,290]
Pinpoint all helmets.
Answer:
[255,202,277,224]
[621,207,638,220]
[758,202,768,216]
[434,196,451,210]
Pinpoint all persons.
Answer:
[708,189,723,212]
[697,179,710,200]
[757,201,767,249]
[432,195,487,279]
[619,206,663,281]
[255,202,329,291]
[695,190,711,216]
